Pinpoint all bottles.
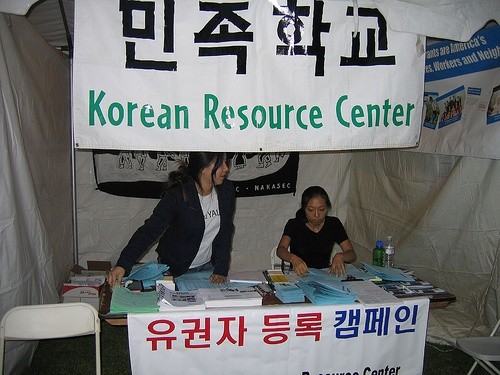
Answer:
[384,236,395,268]
[373,240,384,267]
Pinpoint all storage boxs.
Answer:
[57,260,112,314]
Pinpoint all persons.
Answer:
[108,151,236,288]
[277,186,357,278]
[442,95,461,121]
[424,96,438,124]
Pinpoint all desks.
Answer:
[99,263,457,375]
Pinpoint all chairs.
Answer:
[0,302,101,375]
[457,320,500,375]
[270,243,343,269]
